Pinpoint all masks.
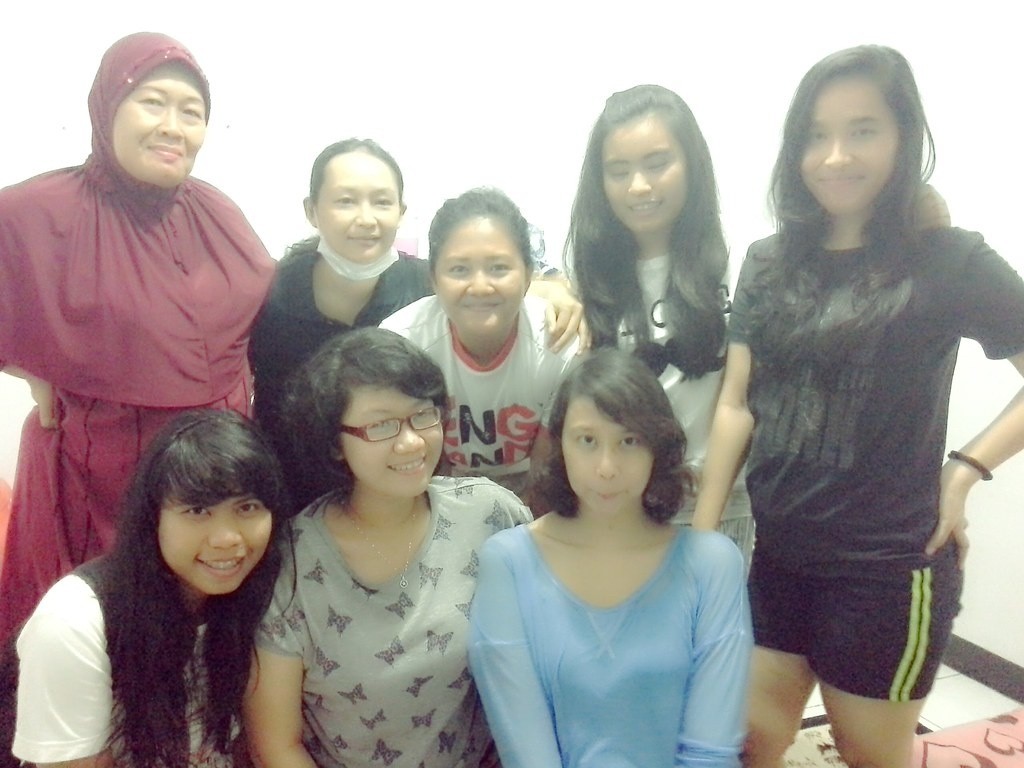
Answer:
[318,231,399,280]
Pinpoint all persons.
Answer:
[563,83,953,582]
[367,188,586,522]
[693,39,1024,768]
[243,136,597,481]
[236,326,540,768]
[0,29,277,680]
[467,346,753,767]
[2,405,300,768]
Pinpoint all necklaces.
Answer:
[341,500,422,591]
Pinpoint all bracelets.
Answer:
[948,446,998,484]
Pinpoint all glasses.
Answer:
[340,405,445,443]
